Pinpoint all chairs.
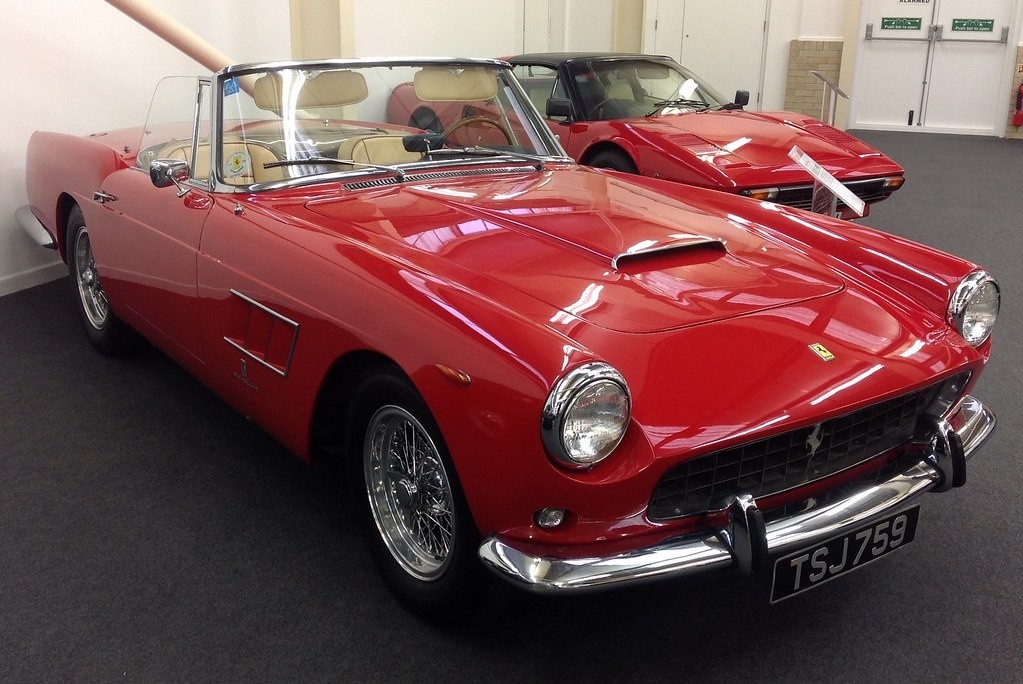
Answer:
[604,84,635,102]
[338,134,427,172]
[157,135,285,186]
[529,86,567,121]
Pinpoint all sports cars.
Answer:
[388,48,907,227]
[13,50,1003,626]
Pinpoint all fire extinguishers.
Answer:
[1012,81,1023,129]
